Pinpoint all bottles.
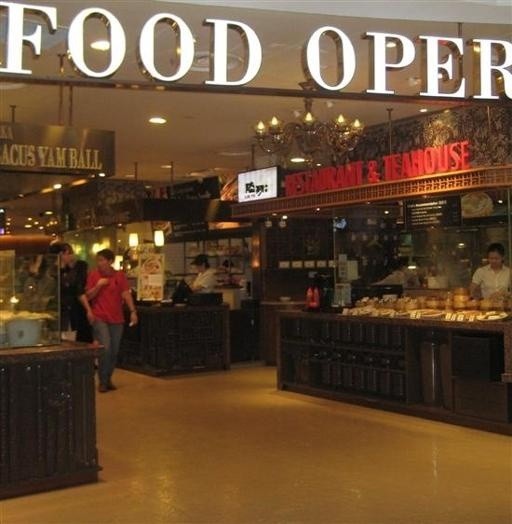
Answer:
[306,285,332,308]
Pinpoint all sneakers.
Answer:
[98,383,117,392]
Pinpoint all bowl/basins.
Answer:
[278,297,292,301]
[144,259,162,275]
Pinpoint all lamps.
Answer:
[251,77,365,175]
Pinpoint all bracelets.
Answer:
[130,308,137,313]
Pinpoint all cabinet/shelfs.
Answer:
[0,233,112,503]
[182,235,245,276]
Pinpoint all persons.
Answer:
[84,248,139,392]
[188,254,215,294]
[470,242,511,300]
[368,260,408,287]
[15,242,95,342]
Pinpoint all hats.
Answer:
[190,254,208,265]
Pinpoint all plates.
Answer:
[348,290,508,322]
